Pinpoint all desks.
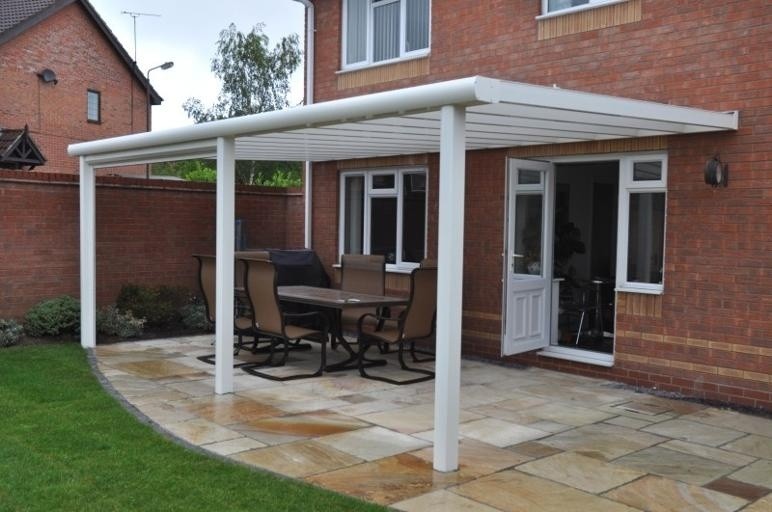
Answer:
[146,60,174,179]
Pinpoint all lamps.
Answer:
[331,255,387,351]
[358,266,436,385]
[558,280,588,343]
[194,252,260,367]
[235,251,287,351]
[240,258,330,381]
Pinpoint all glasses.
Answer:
[704,153,728,187]
